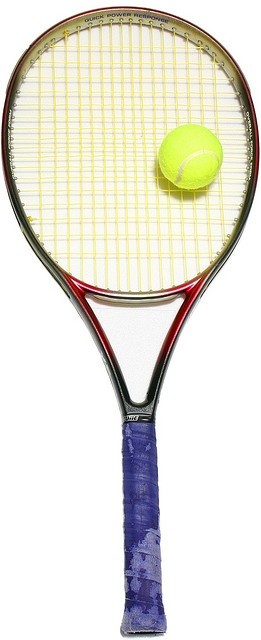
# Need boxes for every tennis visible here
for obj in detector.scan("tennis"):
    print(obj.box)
[159,122,221,188]
